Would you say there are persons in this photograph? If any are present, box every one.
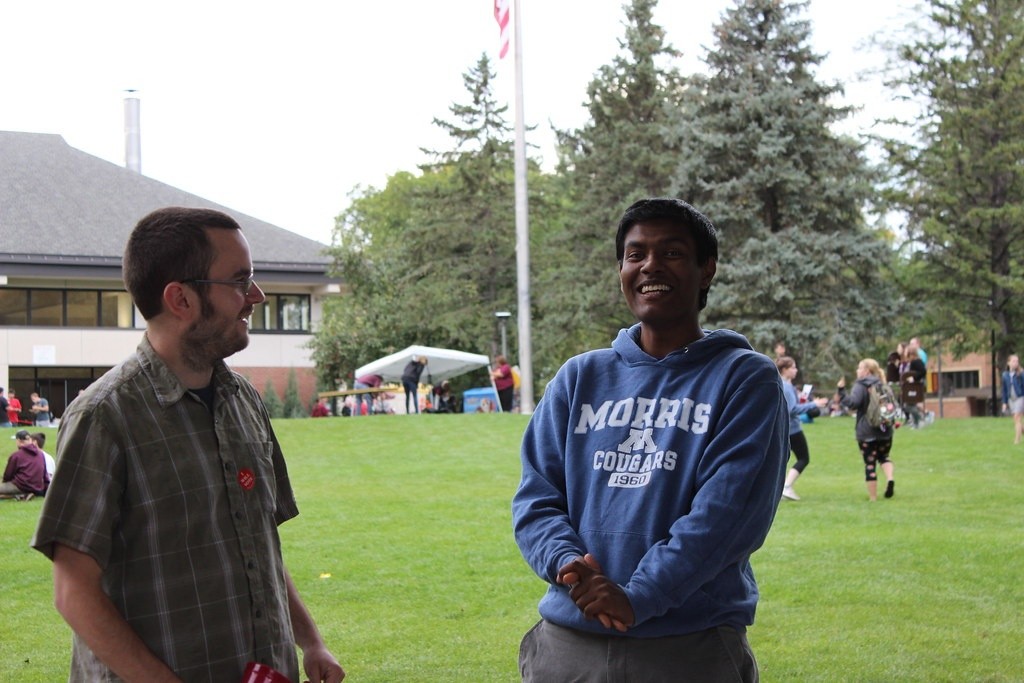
[355,375,383,416]
[512,198,788,683]
[887,338,935,429]
[31,393,50,426]
[829,395,846,416]
[401,357,427,414]
[0,430,55,500]
[33,207,347,683]
[838,359,894,501]
[773,343,827,499]
[313,397,332,417]
[0,387,11,427]
[433,380,450,410]
[489,356,514,412]
[1001,355,1024,443]
[6,389,22,426]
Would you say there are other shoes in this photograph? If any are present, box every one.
[885,480,895,498]
[783,487,799,501]
[909,421,921,430]
[927,412,935,425]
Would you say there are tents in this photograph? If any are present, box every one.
[355,345,503,412]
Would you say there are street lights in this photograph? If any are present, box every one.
[494,311,512,361]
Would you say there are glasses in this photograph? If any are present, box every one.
[185,274,253,296]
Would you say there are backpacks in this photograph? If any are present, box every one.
[865,382,899,433]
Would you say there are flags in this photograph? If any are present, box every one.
[493,0,510,60]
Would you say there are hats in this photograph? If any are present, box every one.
[12,430,31,441]
